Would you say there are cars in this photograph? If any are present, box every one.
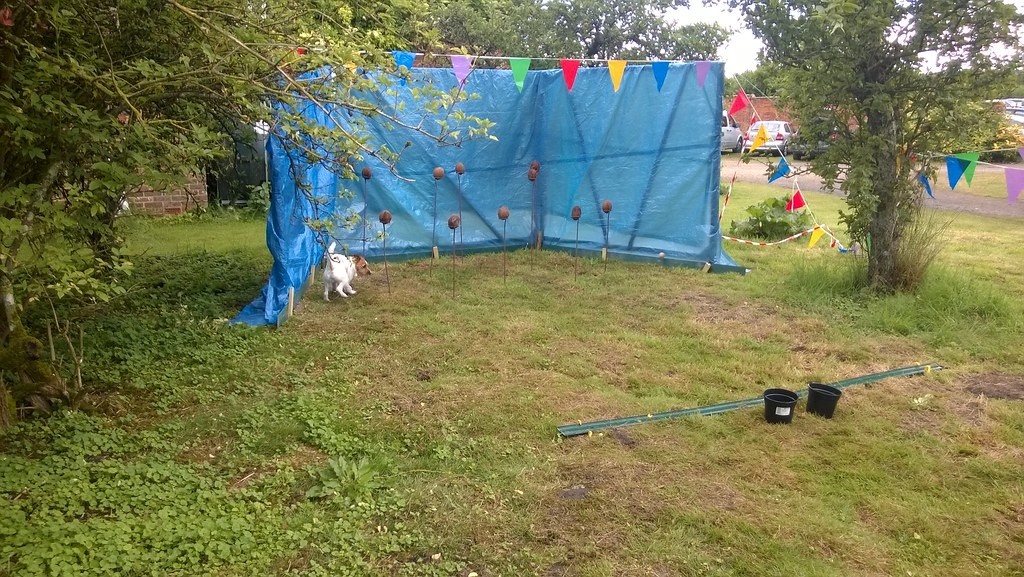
[985,98,1024,116]
[720,110,744,155]
[744,121,800,157]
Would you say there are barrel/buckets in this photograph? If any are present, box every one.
[764,388,799,424]
[806,384,842,419]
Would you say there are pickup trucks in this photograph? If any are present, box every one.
[792,117,853,160]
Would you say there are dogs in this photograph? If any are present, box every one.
[323,242,372,301]
